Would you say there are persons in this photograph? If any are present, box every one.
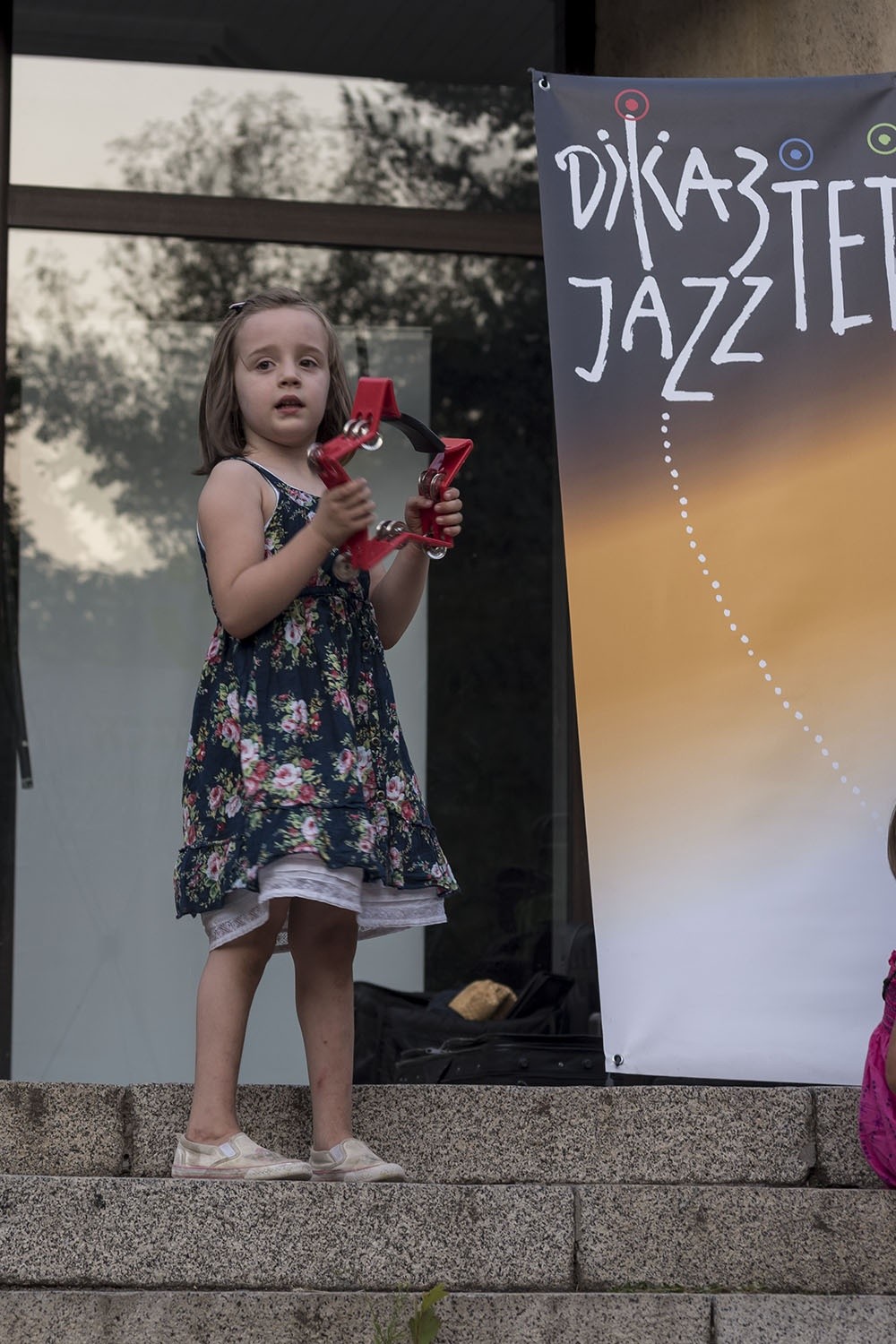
[170,285,462,1182]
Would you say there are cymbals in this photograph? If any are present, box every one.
[306,377,473,571]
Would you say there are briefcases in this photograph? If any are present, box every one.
[395,1032,826,1087]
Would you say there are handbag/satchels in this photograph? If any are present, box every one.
[353,969,590,1090]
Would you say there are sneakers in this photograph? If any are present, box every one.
[308,1138,406,1182]
[170,1133,313,1182]
